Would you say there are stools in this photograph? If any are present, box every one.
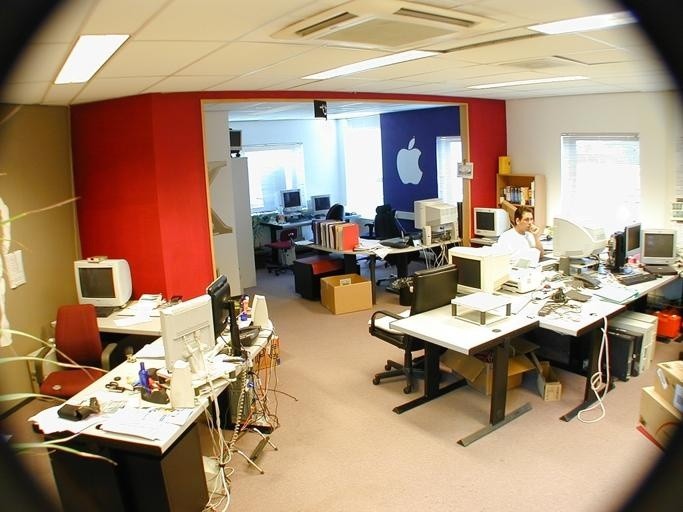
[263,241,291,276]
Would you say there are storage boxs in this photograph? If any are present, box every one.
[537,361,561,402]
[440,338,540,396]
[636,386,683,464]
[321,273,372,314]
[655,360,682,413]
[251,336,280,371]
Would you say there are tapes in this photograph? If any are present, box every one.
[150,389,159,396]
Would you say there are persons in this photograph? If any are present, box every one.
[498,206,544,260]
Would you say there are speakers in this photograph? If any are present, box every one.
[451,221,458,240]
[251,294,268,327]
[423,226,432,245]
[314,100,327,118]
[170,360,196,408]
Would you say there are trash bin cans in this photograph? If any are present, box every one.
[399,276,417,307]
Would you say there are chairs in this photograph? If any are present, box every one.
[368,263,458,394]
[326,204,345,221]
[365,204,413,285]
[34,304,120,400]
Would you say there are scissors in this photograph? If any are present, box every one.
[105,381,122,390]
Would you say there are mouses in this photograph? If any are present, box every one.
[656,274,662,277]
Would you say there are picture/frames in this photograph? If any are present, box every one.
[457,162,473,179]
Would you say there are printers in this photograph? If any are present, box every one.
[502,248,542,294]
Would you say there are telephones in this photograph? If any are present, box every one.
[573,273,602,290]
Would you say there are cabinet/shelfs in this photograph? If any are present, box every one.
[496,174,546,227]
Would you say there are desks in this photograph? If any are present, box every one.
[260,215,359,268]
[295,235,462,305]
[50,301,164,337]
[33,317,275,512]
[488,263,683,422]
[390,304,539,447]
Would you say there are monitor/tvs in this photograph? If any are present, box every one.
[414,197,457,232]
[205,274,246,359]
[640,228,677,265]
[229,129,242,151]
[553,217,608,259]
[279,189,302,213]
[307,194,331,216]
[448,245,512,294]
[73,258,132,308]
[474,207,510,241]
[616,223,641,273]
[160,294,217,379]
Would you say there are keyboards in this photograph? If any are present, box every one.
[96,305,113,318]
[621,273,657,287]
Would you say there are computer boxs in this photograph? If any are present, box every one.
[576,310,660,382]
[26,342,62,399]
[278,246,296,265]
